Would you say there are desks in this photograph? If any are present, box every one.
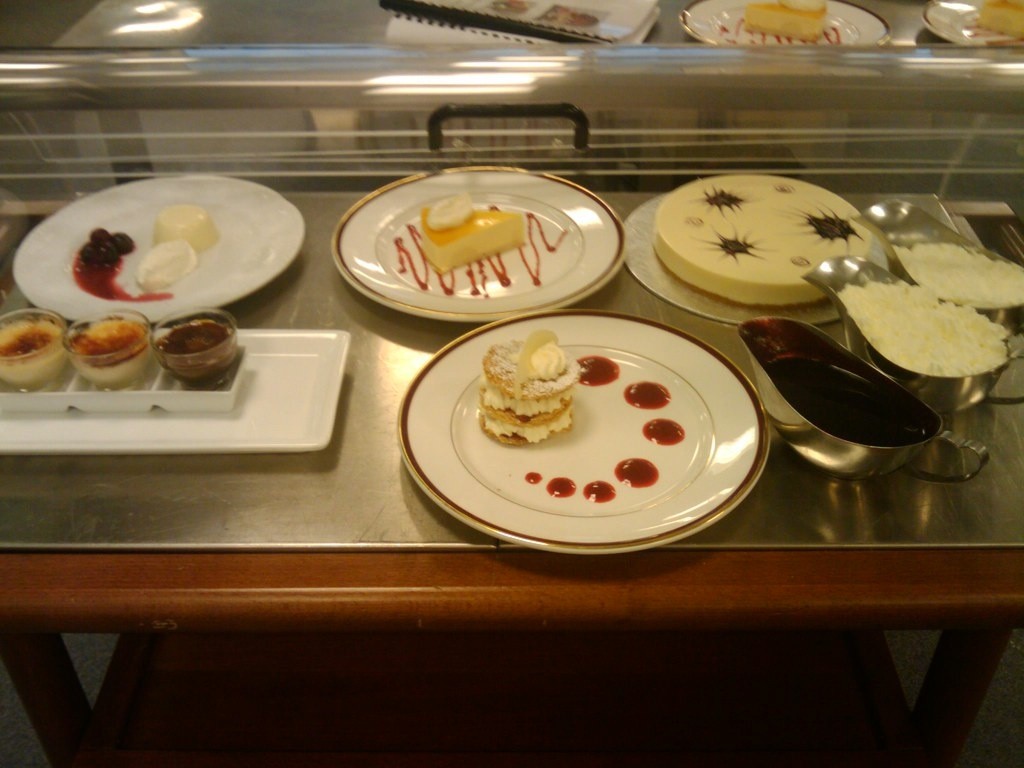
[1,549,1024,768]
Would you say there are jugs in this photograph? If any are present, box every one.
[737,317,989,483]
[851,199,1024,333]
[803,256,1024,426]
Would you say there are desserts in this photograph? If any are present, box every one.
[975,0,1024,40]
[479,328,580,446]
[136,204,219,290]
[417,192,527,275]
[745,0,829,43]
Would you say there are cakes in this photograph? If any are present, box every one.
[651,174,876,312]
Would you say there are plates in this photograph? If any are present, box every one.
[399,309,771,554]
[332,166,627,323]
[13,177,304,322]
[0,327,350,453]
[679,0,891,51]
[921,0,1024,47]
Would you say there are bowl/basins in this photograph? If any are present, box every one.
[61,308,150,389]
[151,306,237,385]
[0,308,67,387]
[0,344,246,413]
